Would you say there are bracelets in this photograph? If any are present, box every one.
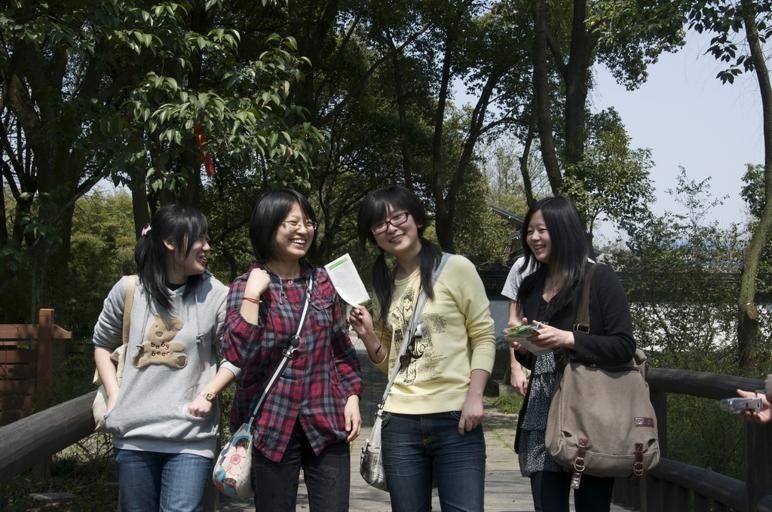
[242,296,263,305]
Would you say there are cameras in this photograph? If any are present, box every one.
[720,397,763,414]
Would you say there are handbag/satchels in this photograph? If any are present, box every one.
[89,343,129,428]
[544,260,661,480]
[359,409,441,494]
[209,420,256,501]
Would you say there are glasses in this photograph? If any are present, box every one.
[370,208,413,235]
[279,217,320,231]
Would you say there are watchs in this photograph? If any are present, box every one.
[200,390,216,404]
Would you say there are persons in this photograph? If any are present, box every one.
[346,183,497,512]
[506,195,636,512]
[219,186,363,512]
[498,252,595,402]
[92,205,241,512]
[735,373,772,430]
